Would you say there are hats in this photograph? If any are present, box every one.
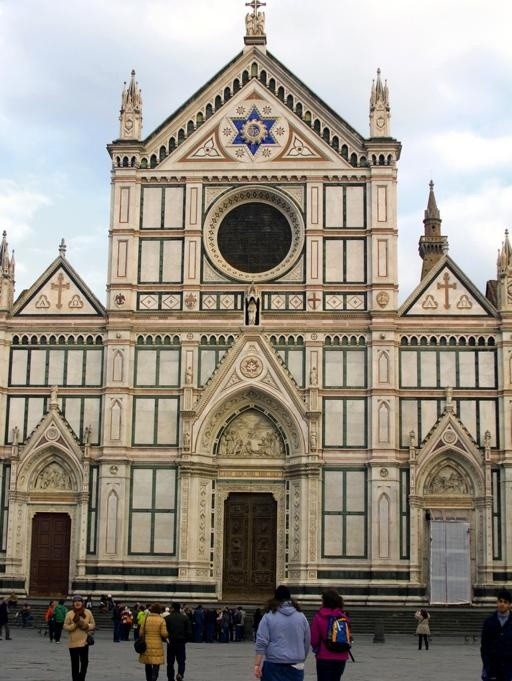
[73,595,82,601]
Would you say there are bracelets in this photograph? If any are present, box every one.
[255,663,262,667]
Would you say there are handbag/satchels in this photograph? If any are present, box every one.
[134,637,146,653]
[86,637,94,646]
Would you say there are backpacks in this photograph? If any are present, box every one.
[326,613,352,653]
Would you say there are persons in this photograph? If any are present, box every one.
[163,604,191,681]
[62,595,96,681]
[43,593,264,641]
[139,602,171,681]
[7,591,19,607]
[480,589,511,681]
[415,607,431,649]
[310,590,352,680]
[0,598,12,642]
[17,602,35,628]
[252,585,311,681]
